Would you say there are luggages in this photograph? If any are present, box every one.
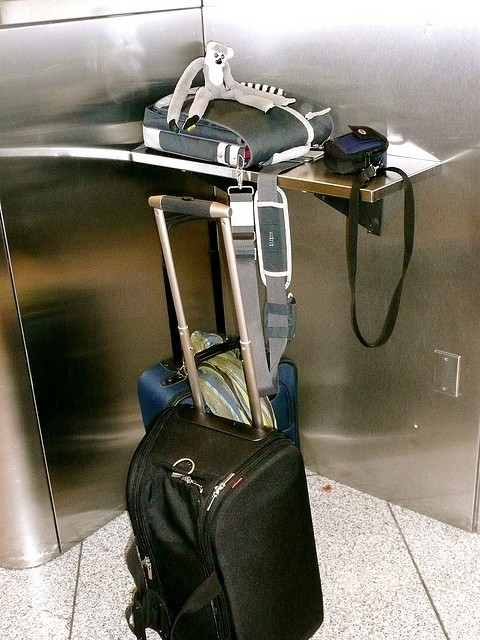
[126,195,324,639]
[137,210,300,449]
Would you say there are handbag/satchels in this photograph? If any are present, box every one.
[141,83,333,165]
[323,125,390,174]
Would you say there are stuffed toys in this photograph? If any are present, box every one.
[166,42,286,133]
